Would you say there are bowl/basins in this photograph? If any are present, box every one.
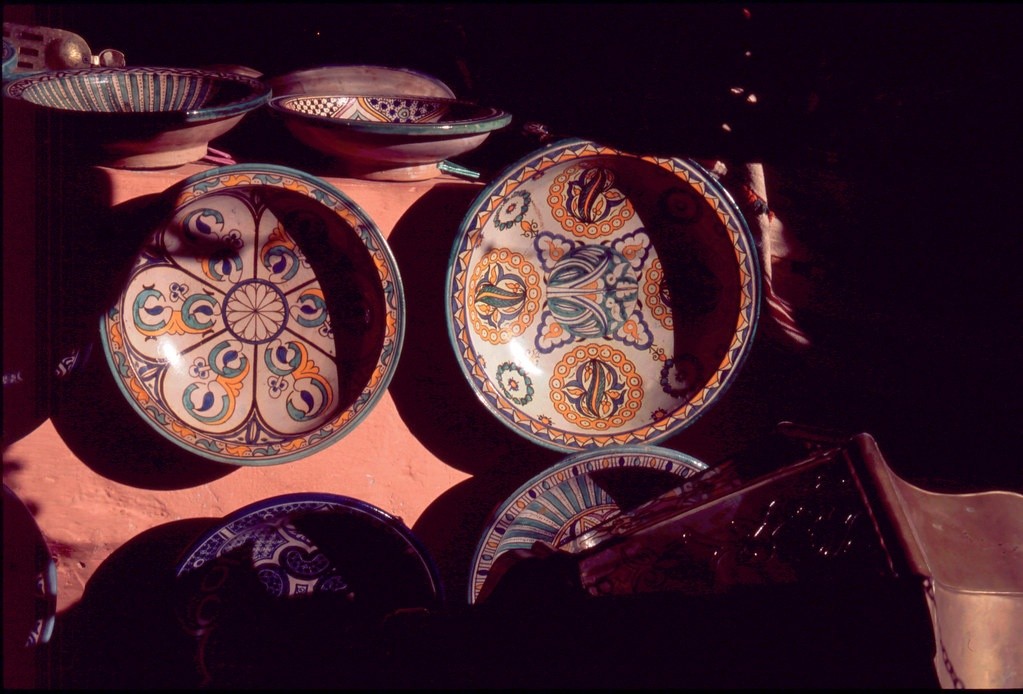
[1,65,271,170]
[273,64,456,99]
[1,480,54,693]
[269,91,514,184]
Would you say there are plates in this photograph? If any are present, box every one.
[99,162,407,468]
[167,492,444,694]
[444,138,763,455]
[466,443,711,605]
